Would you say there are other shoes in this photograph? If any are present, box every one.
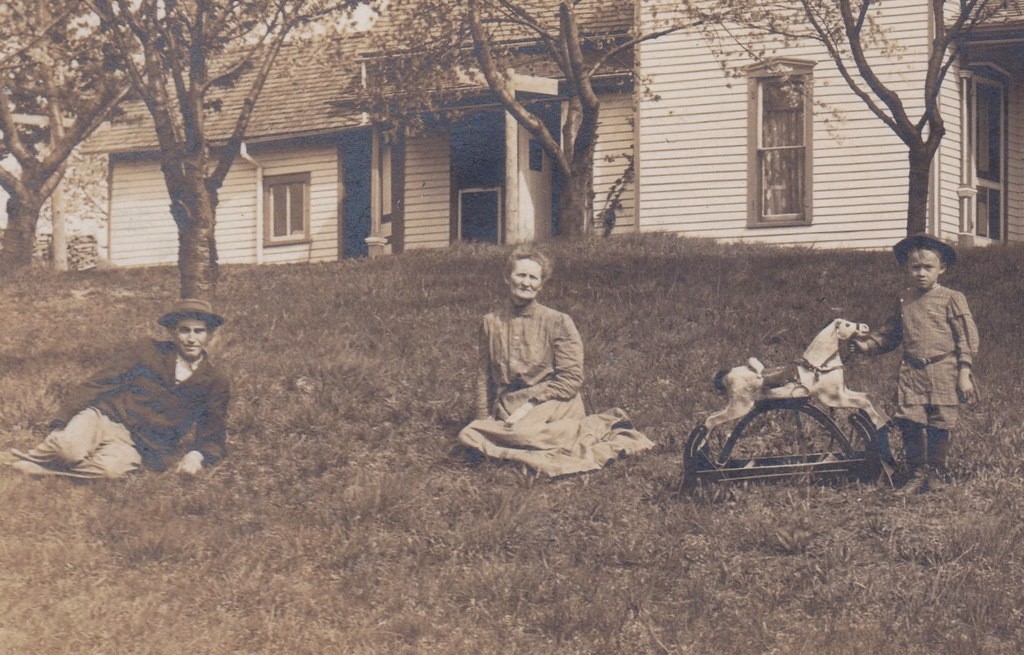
[924,463,949,496]
[897,475,925,494]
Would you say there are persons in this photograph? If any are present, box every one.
[455,249,656,477]
[0,300,230,478]
[848,233,979,496]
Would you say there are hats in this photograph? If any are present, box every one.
[893,232,956,267]
[157,298,224,327]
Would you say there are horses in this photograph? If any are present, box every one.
[697,317,885,449]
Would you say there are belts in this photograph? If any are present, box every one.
[903,348,959,369]
